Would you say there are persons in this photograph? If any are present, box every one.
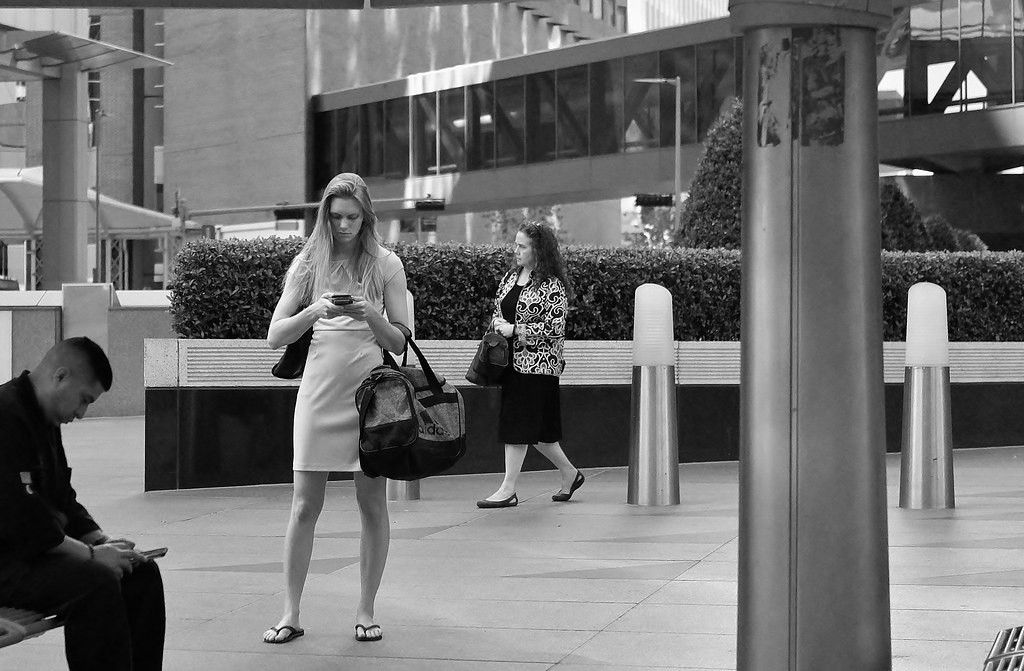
[0,336,166,671]
[473,219,588,511]
[260,171,409,638]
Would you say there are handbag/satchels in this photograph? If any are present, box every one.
[271,253,314,380]
[355,322,465,482]
[465,318,509,387]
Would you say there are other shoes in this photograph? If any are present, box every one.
[552,469,585,502]
[477,492,518,508]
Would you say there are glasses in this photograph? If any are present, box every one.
[531,220,543,239]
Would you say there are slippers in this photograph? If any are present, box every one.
[263,626,304,643]
[355,623,382,641]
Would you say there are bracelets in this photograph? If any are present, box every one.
[512,323,517,336]
[93,535,110,546]
[83,543,95,565]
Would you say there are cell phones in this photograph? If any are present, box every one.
[332,295,353,305]
[131,548,168,563]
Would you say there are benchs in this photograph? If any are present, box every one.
[983,626,1024,671]
[0,605,64,648]
